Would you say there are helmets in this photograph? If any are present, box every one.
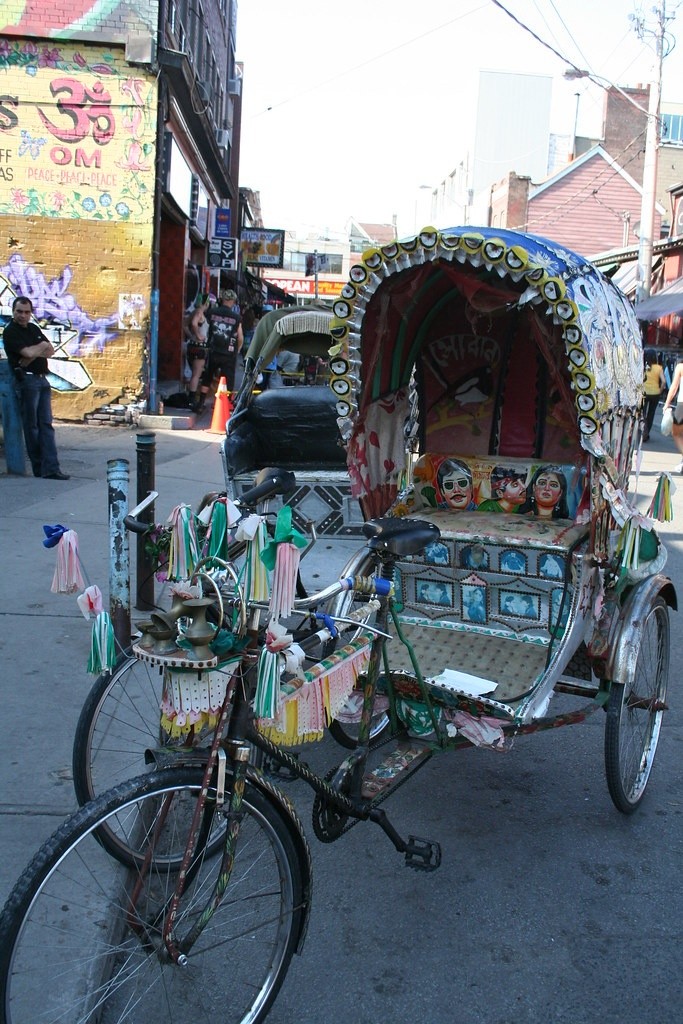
[261,304,273,312]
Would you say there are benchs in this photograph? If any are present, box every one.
[226,383,386,538]
[393,448,595,580]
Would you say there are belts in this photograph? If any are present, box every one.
[25,371,44,378]
[189,341,207,348]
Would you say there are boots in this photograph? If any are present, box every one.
[185,391,201,414]
[198,392,206,409]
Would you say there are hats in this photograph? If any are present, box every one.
[222,289,237,301]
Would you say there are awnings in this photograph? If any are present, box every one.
[635,276,683,319]
[610,254,662,296]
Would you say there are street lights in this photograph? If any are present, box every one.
[565,66,661,308]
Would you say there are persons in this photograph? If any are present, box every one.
[663,360,683,452]
[243,305,327,391]
[3,296,72,480]
[641,349,666,442]
[197,289,244,411]
[184,294,209,405]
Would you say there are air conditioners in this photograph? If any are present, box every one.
[197,81,214,102]
[227,80,241,97]
[213,129,229,151]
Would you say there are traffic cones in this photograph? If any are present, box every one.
[203,376,232,436]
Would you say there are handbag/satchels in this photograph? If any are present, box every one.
[660,408,673,437]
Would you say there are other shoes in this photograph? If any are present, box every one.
[43,471,71,480]
[643,435,649,443]
[34,473,41,477]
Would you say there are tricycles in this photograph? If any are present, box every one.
[0,225,678,1023]
[71,299,392,873]
